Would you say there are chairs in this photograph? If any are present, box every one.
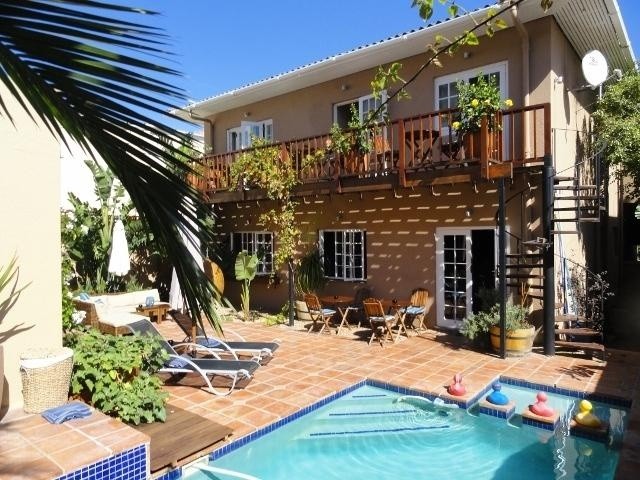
[302,294,337,337]
[166,310,278,365]
[126,318,261,396]
[362,299,395,348]
[341,287,370,329]
[399,287,429,337]
[369,127,464,172]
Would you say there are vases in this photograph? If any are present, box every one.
[463,132,483,166]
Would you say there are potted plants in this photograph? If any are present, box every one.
[457,302,533,358]
[292,248,327,320]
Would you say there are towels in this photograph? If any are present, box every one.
[42,401,91,424]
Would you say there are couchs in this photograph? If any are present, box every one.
[73,288,160,339]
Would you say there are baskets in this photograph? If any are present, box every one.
[20,345,75,415]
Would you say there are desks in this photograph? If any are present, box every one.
[318,295,355,334]
[371,297,411,333]
[134,305,171,323]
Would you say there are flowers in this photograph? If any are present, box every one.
[450,71,514,133]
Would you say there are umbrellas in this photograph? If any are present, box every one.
[109,219,131,279]
[170,196,205,344]
